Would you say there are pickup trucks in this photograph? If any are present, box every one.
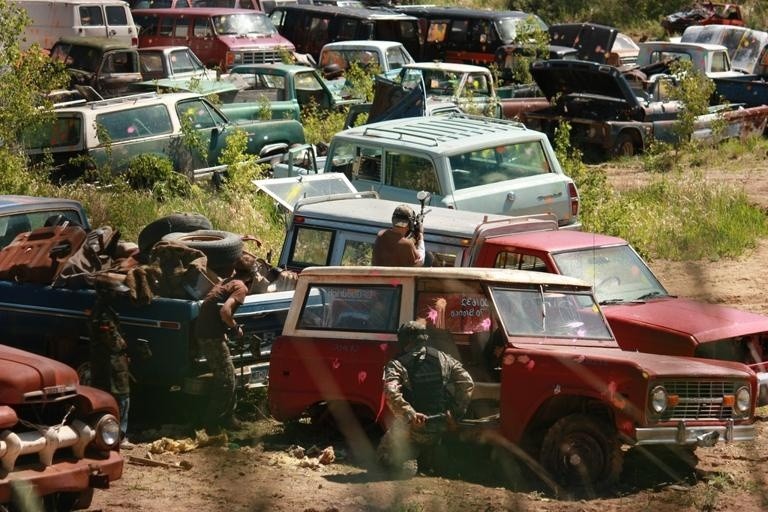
[1,192,331,419]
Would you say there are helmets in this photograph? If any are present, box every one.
[397,320,429,351]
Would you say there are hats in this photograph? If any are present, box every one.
[393,204,414,229]
[96,275,130,294]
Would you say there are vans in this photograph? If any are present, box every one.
[269,112,584,234]
[0,2,768,191]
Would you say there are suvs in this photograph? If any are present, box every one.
[0,340,127,512]
[265,265,761,494]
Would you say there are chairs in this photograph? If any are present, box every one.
[427,328,501,415]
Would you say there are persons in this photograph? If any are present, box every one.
[374,321,474,479]
[487,328,505,383]
[87,288,130,440]
[195,254,255,430]
[372,206,426,267]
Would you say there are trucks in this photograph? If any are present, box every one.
[248,173,767,409]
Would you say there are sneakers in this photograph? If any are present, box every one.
[119,438,134,449]
[225,417,251,430]
[400,445,421,480]
[212,426,236,437]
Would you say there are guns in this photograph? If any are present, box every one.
[416,210,432,225]
